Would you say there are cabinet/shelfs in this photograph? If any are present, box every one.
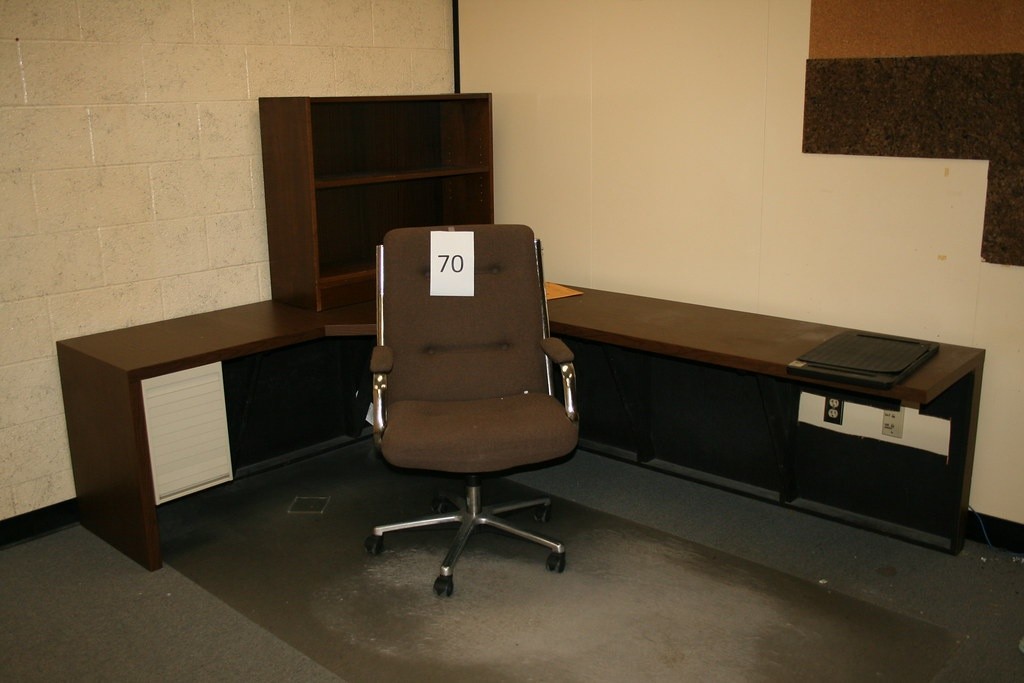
[260,92,494,313]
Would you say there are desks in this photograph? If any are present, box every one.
[55,282,986,573]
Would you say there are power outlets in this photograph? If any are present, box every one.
[823,395,845,426]
[881,405,906,439]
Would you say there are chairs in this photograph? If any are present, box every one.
[364,225,581,600]
